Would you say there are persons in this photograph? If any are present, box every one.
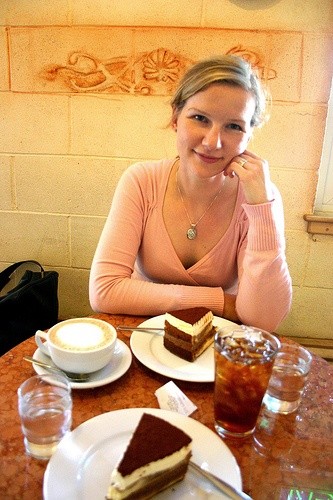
[89,57,292,333]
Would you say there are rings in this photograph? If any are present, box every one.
[239,159,248,167]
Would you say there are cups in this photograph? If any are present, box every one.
[262,342,313,415]
[35,317,117,373]
[213,324,281,441]
[17,375,73,460]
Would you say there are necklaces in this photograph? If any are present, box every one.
[175,164,225,241]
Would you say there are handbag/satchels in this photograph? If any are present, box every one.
[0,260,59,356]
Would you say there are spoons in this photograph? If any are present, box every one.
[24,356,90,380]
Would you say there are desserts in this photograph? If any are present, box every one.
[104,412,193,500]
[163,306,218,362]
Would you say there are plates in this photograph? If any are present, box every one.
[130,315,240,383]
[32,339,132,390]
[43,408,242,500]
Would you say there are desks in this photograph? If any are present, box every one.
[0,312,333,500]
